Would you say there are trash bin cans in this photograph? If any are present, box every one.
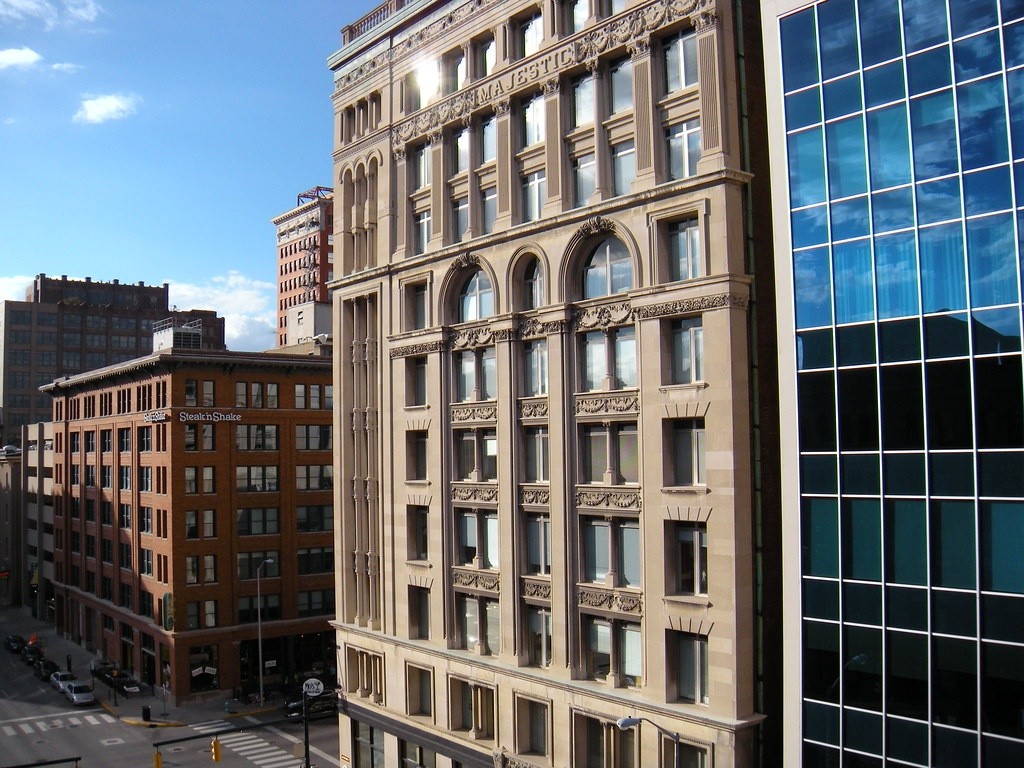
[141,706,151,722]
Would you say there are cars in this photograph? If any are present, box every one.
[64,681,94,706]
[285,691,339,718]
[5,635,25,653]
[19,645,44,666]
[33,659,60,681]
[50,670,80,693]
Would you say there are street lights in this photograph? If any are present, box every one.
[617,716,681,768]
[257,558,273,707]
